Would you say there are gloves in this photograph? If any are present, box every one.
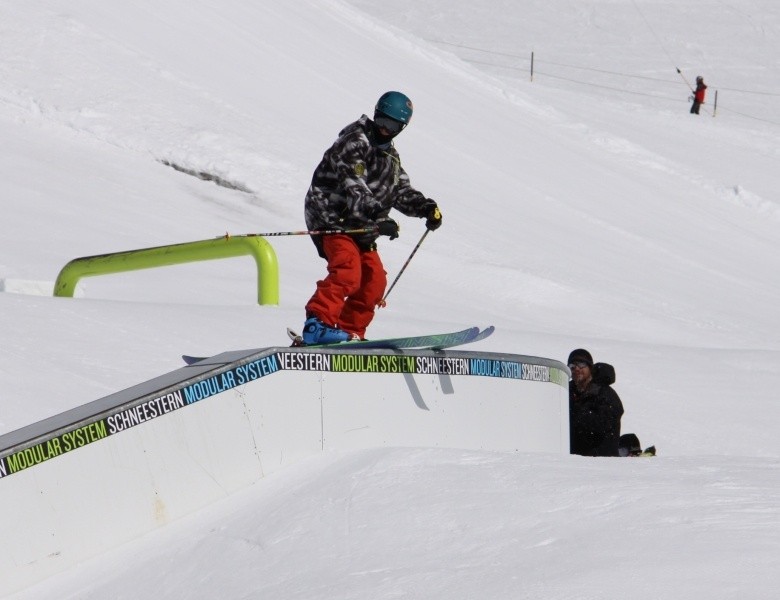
[375,216,400,240]
[424,205,443,232]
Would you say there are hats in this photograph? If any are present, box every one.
[568,348,593,368]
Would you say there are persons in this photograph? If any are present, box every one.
[690,76,705,113]
[300,92,447,345]
[570,348,623,458]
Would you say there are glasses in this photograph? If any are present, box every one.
[568,361,588,368]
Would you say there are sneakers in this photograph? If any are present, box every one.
[303,318,353,345]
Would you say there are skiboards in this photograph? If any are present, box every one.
[181,323,496,364]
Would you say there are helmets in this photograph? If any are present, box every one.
[375,90,414,124]
[696,75,703,81]
